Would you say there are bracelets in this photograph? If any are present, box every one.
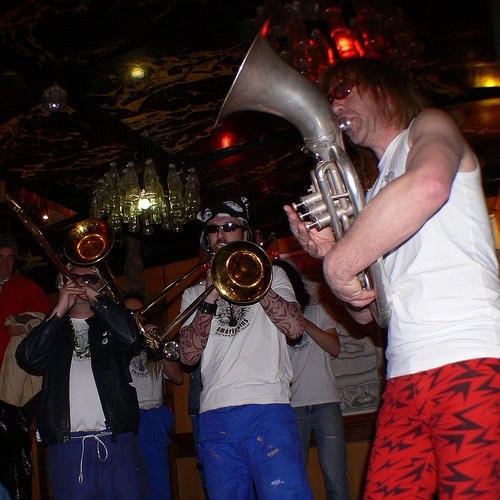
[196,300,218,315]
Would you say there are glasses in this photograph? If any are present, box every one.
[126,307,144,317]
[205,222,246,234]
[326,79,368,104]
[65,273,104,285]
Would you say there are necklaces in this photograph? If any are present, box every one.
[68,314,92,359]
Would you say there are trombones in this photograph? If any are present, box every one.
[4,193,125,306]
[129,241,274,350]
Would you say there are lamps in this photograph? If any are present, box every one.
[87,159,201,235]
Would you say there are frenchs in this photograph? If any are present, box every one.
[211,30,392,330]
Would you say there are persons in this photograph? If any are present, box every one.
[0,196,349,500]
[283,55,500,500]
